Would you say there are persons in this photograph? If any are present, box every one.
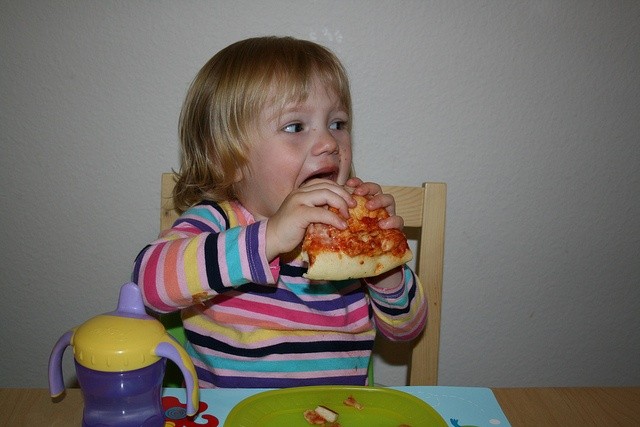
[130,36,428,389]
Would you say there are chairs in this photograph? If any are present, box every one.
[159,172,448,386]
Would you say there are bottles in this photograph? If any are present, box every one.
[46,283,197,425]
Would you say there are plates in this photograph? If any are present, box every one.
[223,386,448,426]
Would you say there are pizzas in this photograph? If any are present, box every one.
[299,195,413,281]
